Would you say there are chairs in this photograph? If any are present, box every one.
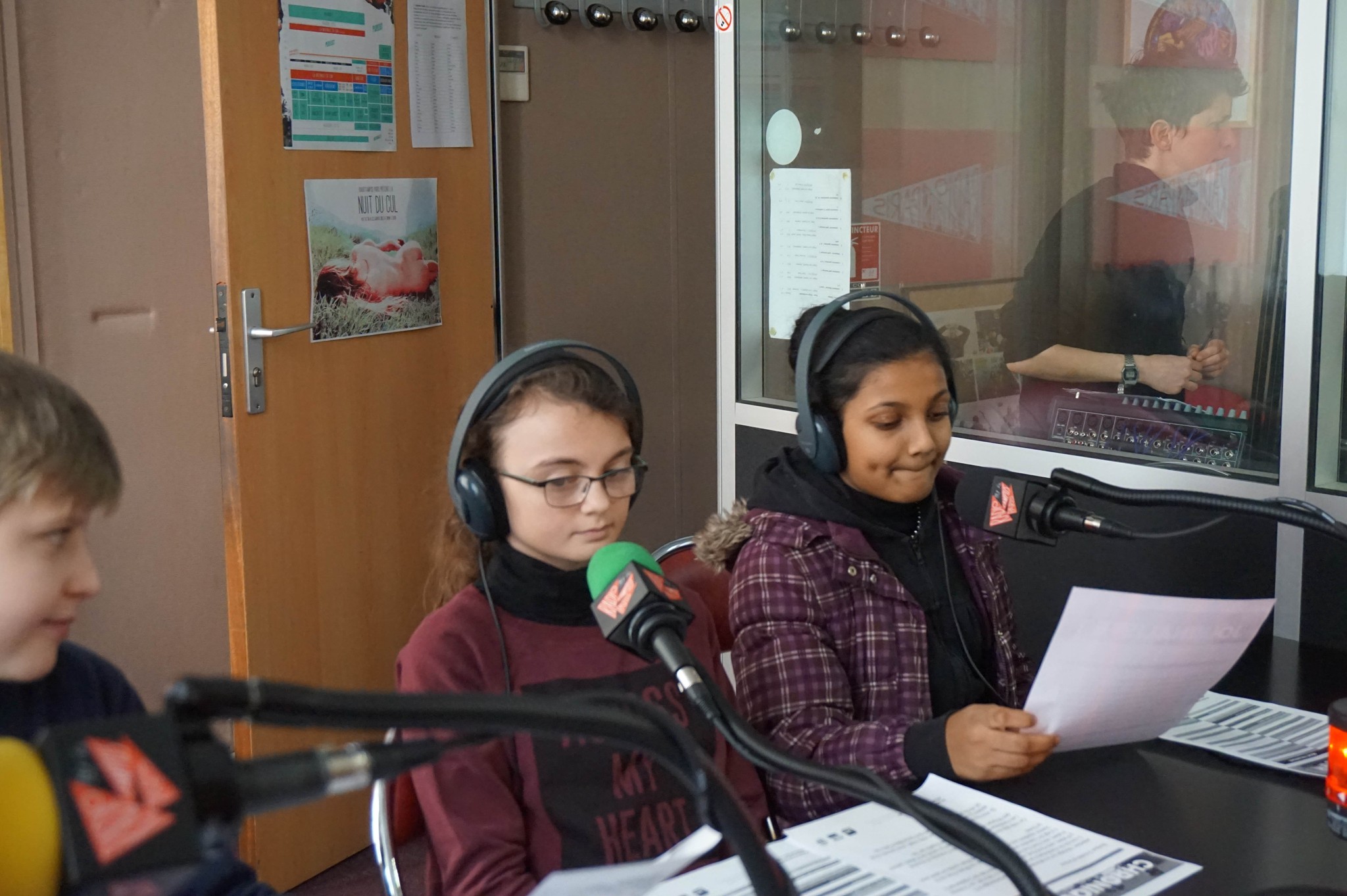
[653,522,736,656]
[367,726,447,896]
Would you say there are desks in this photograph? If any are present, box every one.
[967,686,1347,896]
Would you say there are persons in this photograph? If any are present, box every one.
[392,353,765,896]
[689,302,1060,826]
[317,232,437,318]
[998,49,1249,441]
[0,346,281,896]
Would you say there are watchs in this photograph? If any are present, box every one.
[1122,353,1140,389]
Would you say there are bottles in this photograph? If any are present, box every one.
[1326,697,1347,841]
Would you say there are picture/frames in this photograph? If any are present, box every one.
[899,275,1023,437]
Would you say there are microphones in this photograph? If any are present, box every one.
[0,714,444,896]
[585,541,723,725]
[953,469,1134,548]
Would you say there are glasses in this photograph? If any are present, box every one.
[496,453,649,508]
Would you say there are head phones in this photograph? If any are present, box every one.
[795,290,958,475]
[446,340,643,542]
